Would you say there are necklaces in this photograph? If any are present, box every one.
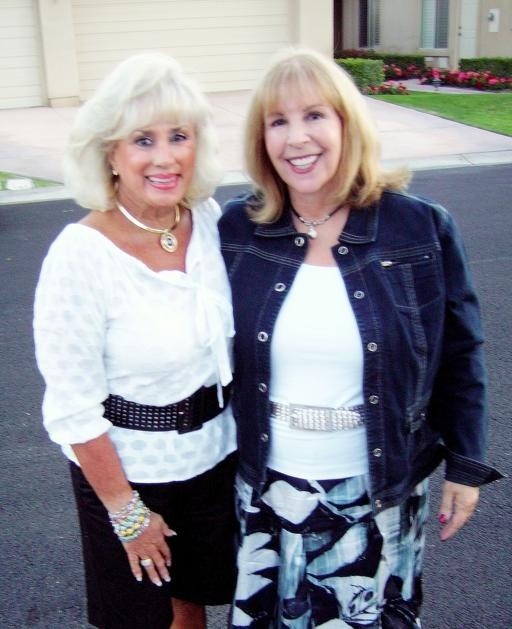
[290,198,347,241]
[111,198,182,254]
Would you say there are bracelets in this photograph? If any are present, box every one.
[104,489,151,541]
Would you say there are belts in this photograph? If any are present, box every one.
[101,380,233,436]
[268,399,367,433]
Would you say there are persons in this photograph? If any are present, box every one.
[211,49,510,628]
[32,62,241,628]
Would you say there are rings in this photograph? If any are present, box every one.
[139,558,153,567]
